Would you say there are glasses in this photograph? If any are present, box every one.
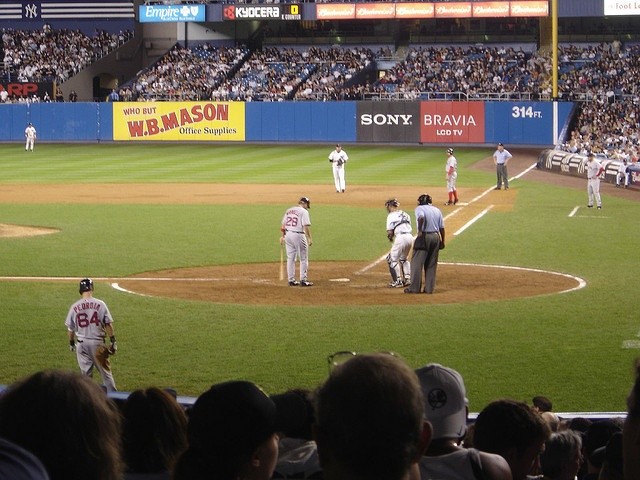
[327,349,398,378]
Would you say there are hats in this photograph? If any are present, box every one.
[414,362,469,441]
[587,153,593,157]
[187,381,307,477]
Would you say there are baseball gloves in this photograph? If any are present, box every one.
[336,157,344,165]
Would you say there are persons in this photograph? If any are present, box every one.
[584,420,622,480]
[275,388,325,479]
[281,197,313,286]
[616,158,631,188]
[1,20,132,102]
[66,277,118,393]
[533,396,552,414]
[445,148,459,206]
[542,412,559,432]
[25,123,36,152]
[557,37,640,102]
[403,195,445,294]
[329,144,349,193]
[105,42,553,101]
[623,359,640,479]
[610,418,624,432]
[169,382,280,480]
[404,362,511,480]
[565,104,640,153]
[318,355,434,480]
[493,142,513,190]
[605,153,640,162]
[570,418,591,432]
[474,399,551,479]
[585,153,604,210]
[591,431,624,480]
[543,431,584,480]
[1,370,123,480]
[111,387,187,479]
[556,420,570,432]
[384,198,415,288]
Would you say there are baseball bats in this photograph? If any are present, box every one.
[280,238,284,283]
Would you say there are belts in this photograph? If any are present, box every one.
[286,230,304,234]
[422,231,437,234]
[401,232,407,234]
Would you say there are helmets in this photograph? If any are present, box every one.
[300,197,310,209]
[336,144,341,147]
[80,278,93,291]
[385,199,399,207]
[447,148,453,155]
[417,194,432,205]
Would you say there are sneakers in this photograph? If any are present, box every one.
[597,206,601,209]
[444,201,453,206]
[404,288,413,293]
[505,187,509,191]
[387,280,404,288]
[494,187,501,190]
[454,199,458,205]
[424,287,432,294]
[402,279,411,286]
[300,281,314,286]
[288,279,300,285]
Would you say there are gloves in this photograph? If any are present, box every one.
[70,344,77,352]
[108,342,117,355]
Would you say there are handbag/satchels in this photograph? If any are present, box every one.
[414,232,426,250]
